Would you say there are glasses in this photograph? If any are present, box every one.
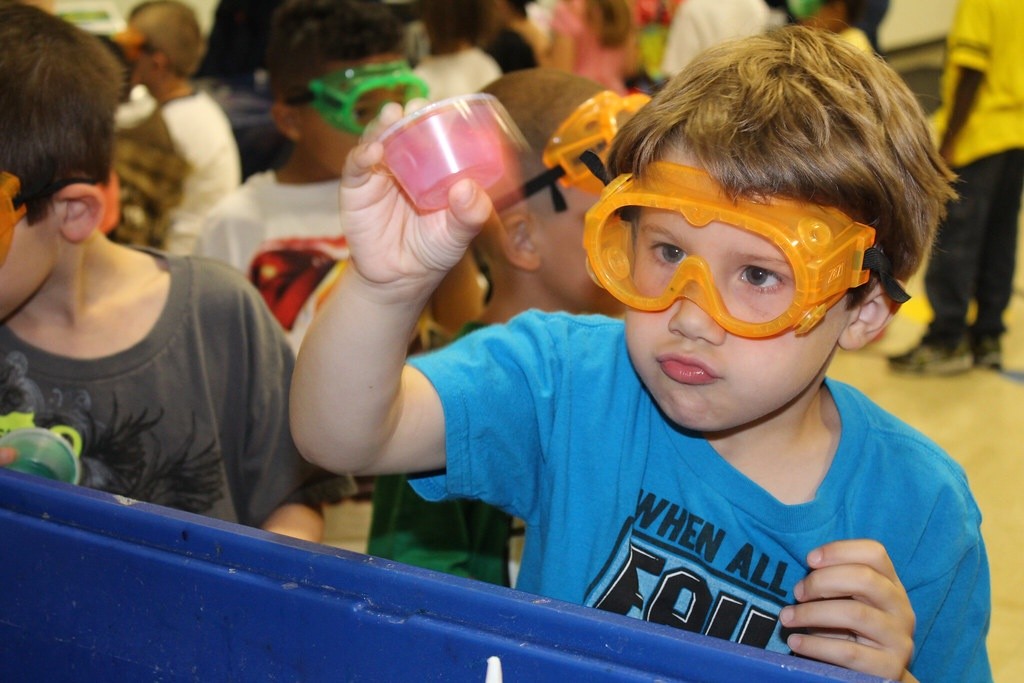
[582,162,911,339]
[492,91,651,215]
[284,58,429,135]
[112,29,191,80]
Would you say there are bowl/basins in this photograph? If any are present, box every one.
[372,92,505,211]
[0,428,80,484]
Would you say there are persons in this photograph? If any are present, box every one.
[289,22,995,683]
[0,0,357,546]
[1,0,1023,377]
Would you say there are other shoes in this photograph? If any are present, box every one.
[891,333,1001,373]
